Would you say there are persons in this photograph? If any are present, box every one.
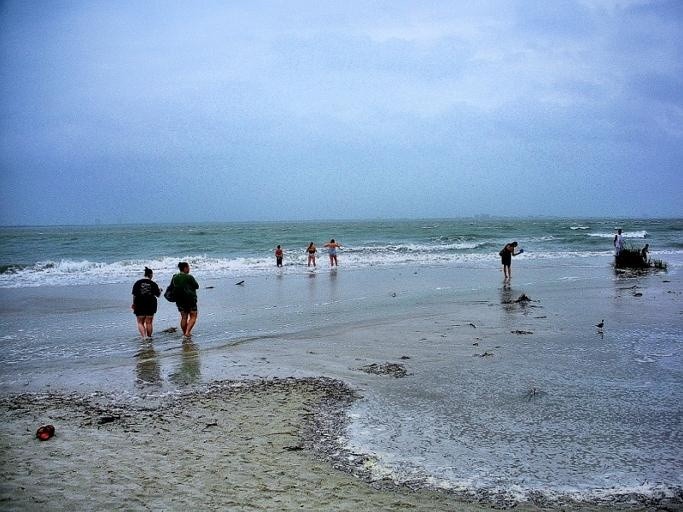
[305,242,317,267]
[133,339,161,387]
[167,338,200,386]
[641,244,649,263]
[499,242,523,278]
[613,228,627,269]
[131,266,163,338]
[503,278,512,291]
[171,262,199,337]
[274,244,283,267]
[323,239,340,266]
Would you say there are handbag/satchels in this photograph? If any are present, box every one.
[164,274,178,302]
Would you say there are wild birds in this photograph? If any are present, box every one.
[593,319,605,332]
